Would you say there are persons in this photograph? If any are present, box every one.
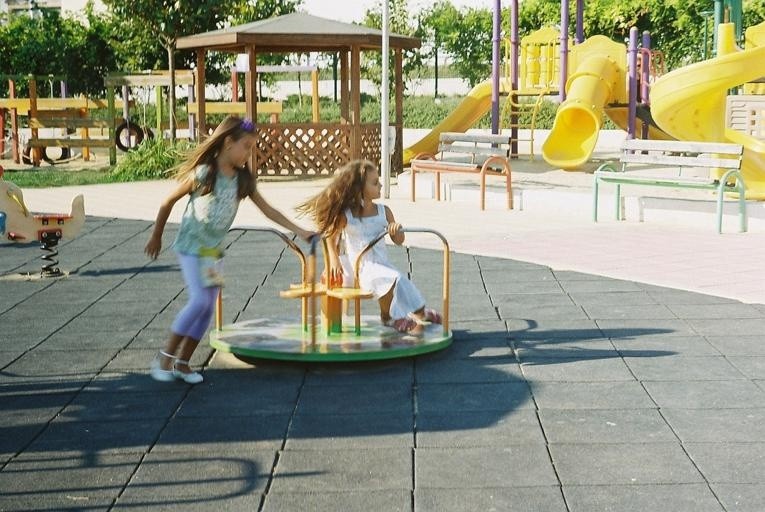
[295,159,444,333]
[634,56,642,103]
[143,113,321,385]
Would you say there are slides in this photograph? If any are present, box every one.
[402,21,765,199]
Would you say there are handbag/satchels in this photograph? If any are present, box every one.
[197,254,225,290]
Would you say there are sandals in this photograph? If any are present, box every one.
[382,315,417,332]
[420,308,443,324]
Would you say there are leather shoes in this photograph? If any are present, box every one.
[173,359,204,384]
[150,350,176,384]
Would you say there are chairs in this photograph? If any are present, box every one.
[0,165,86,280]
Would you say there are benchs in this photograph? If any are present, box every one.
[409,132,514,210]
[593,140,747,233]
